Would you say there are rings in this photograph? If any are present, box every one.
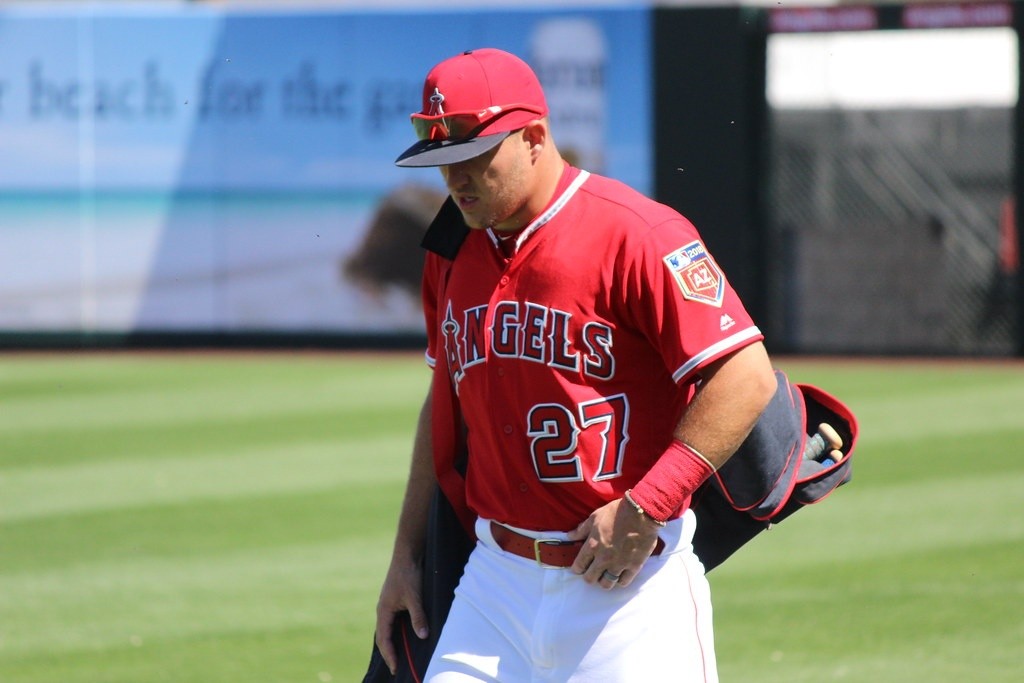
[603,571,620,583]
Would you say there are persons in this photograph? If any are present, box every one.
[372,46,780,683]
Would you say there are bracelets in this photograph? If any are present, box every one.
[624,488,666,527]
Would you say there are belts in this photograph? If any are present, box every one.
[489,521,666,566]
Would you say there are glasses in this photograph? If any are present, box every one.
[410,103,543,142]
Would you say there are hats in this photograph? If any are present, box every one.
[395,49,549,167]
